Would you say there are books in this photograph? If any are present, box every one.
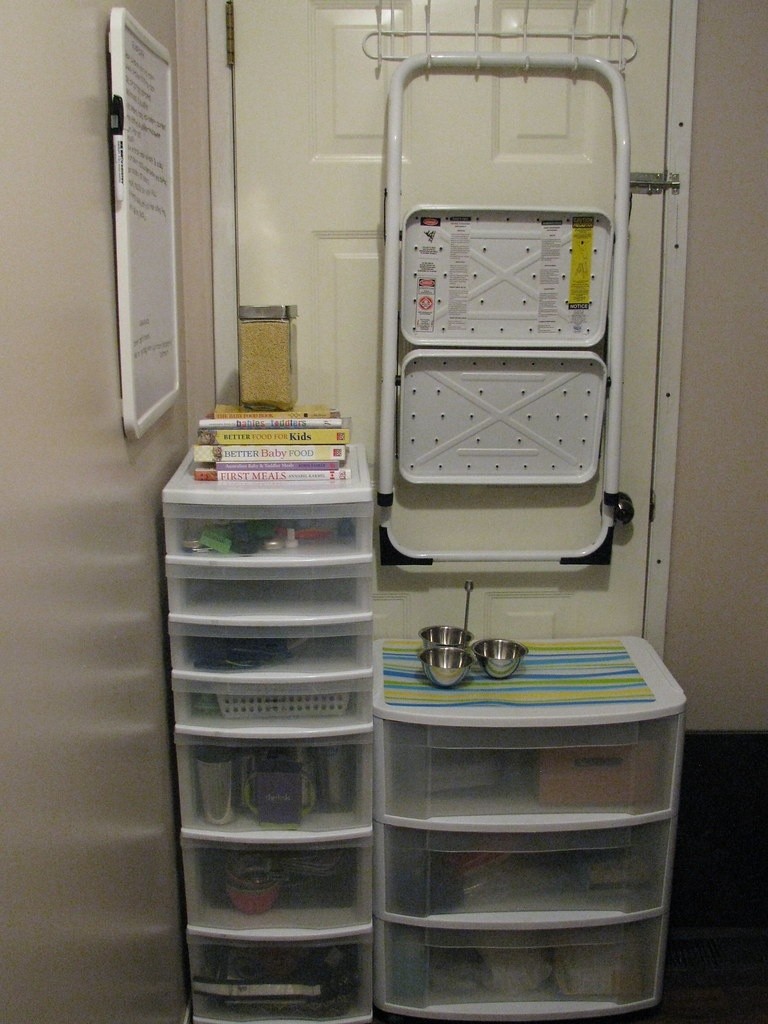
[195,404,353,482]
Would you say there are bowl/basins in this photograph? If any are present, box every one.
[472,638,529,679]
[224,863,277,912]
[417,646,476,688]
[417,625,475,651]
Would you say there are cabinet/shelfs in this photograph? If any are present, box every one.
[373,638,686,1021]
[161,444,377,1024]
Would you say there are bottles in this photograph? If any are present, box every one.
[238,305,298,412]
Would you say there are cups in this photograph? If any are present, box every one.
[194,748,241,826]
[316,744,355,813]
